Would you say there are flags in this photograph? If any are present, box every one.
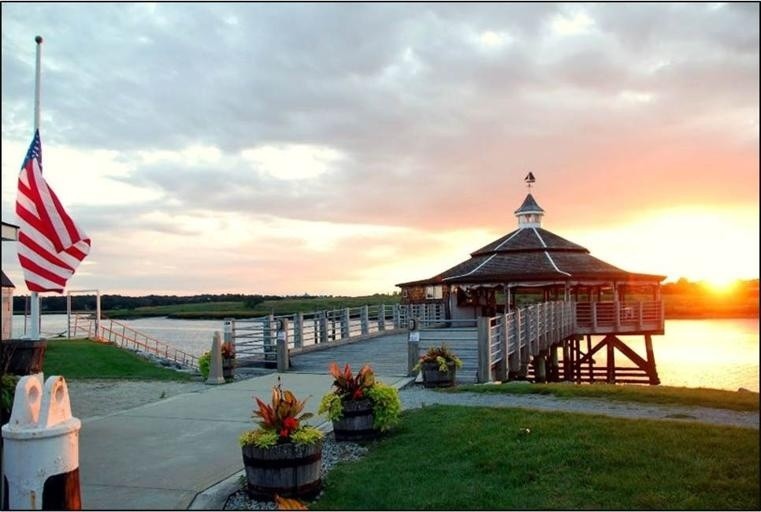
[16,128,93,294]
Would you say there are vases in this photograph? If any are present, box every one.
[421,362,455,389]
[221,358,234,383]
[332,401,372,442]
[241,443,322,502]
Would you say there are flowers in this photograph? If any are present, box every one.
[220,342,238,369]
[411,340,462,373]
[238,375,324,445]
[316,362,400,431]
